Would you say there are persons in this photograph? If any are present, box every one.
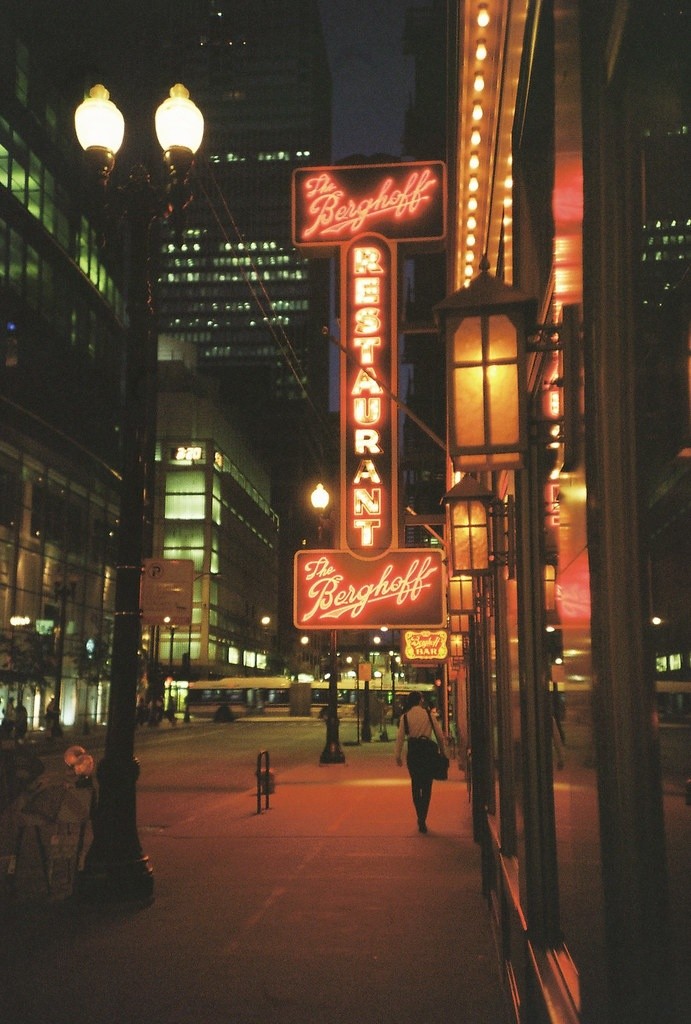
[395,692,446,832]
[0,698,178,743]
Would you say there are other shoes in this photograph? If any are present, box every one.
[418,819,427,832]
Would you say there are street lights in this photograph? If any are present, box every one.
[348,664,360,743]
[309,481,347,764]
[81,636,95,735]
[74,81,205,916]
[373,671,382,732]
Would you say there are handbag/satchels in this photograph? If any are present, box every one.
[429,751,450,781]
[408,735,439,768]
